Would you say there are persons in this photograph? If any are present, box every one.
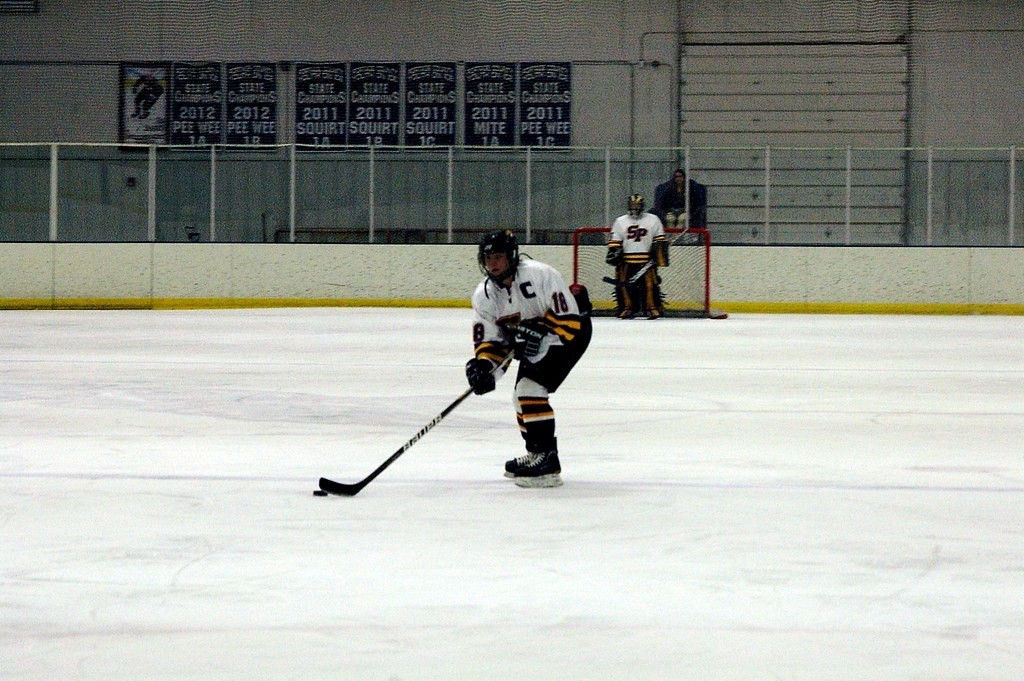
[464,229,592,487]
[664,169,690,241]
[607,195,669,321]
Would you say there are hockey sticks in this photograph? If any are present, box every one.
[602,232,686,287]
[319,348,515,498]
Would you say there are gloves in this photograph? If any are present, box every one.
[510,319,550,359]
[464,358,497,396]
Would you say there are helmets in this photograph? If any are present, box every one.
[475,229,519,280]
[627,193,645,215]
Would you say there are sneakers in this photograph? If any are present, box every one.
[504,451,538,478]
[514,436,563,488]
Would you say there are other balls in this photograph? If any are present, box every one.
[313,490,327,496]
